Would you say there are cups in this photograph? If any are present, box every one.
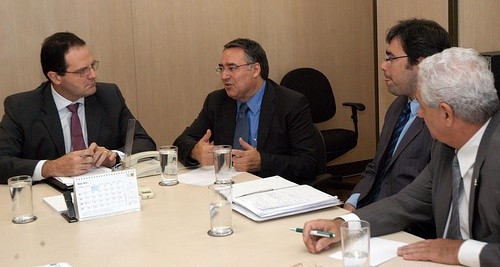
[158,145,178,186]
[340,219,371,267]
[207,183,233,236]
[212,145,233,185]
[7,175,34,224]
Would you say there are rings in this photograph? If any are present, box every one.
[107,150,110,153]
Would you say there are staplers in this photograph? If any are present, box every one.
[61,191,79,224]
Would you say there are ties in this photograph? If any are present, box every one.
[233,103,249,150]
[66,103,87,150]
[446,156,462,240]
[367,102,411,204]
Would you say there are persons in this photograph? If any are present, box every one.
[171,37,324,185]
[344,18,451,240]
[303,46,500,267]
[0,32,156,185]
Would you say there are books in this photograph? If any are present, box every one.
[44,166,113,191]
[215,175,343,222]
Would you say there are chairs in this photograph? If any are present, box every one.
[279,68,364,190]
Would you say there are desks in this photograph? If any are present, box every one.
[0,160,465,267]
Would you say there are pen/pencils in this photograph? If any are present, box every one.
[80,155,87,159]
[289,228,335,238]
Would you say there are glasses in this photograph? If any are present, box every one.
[215,63,256,74]
[384,56,408,61]
[58,60,98,77]
